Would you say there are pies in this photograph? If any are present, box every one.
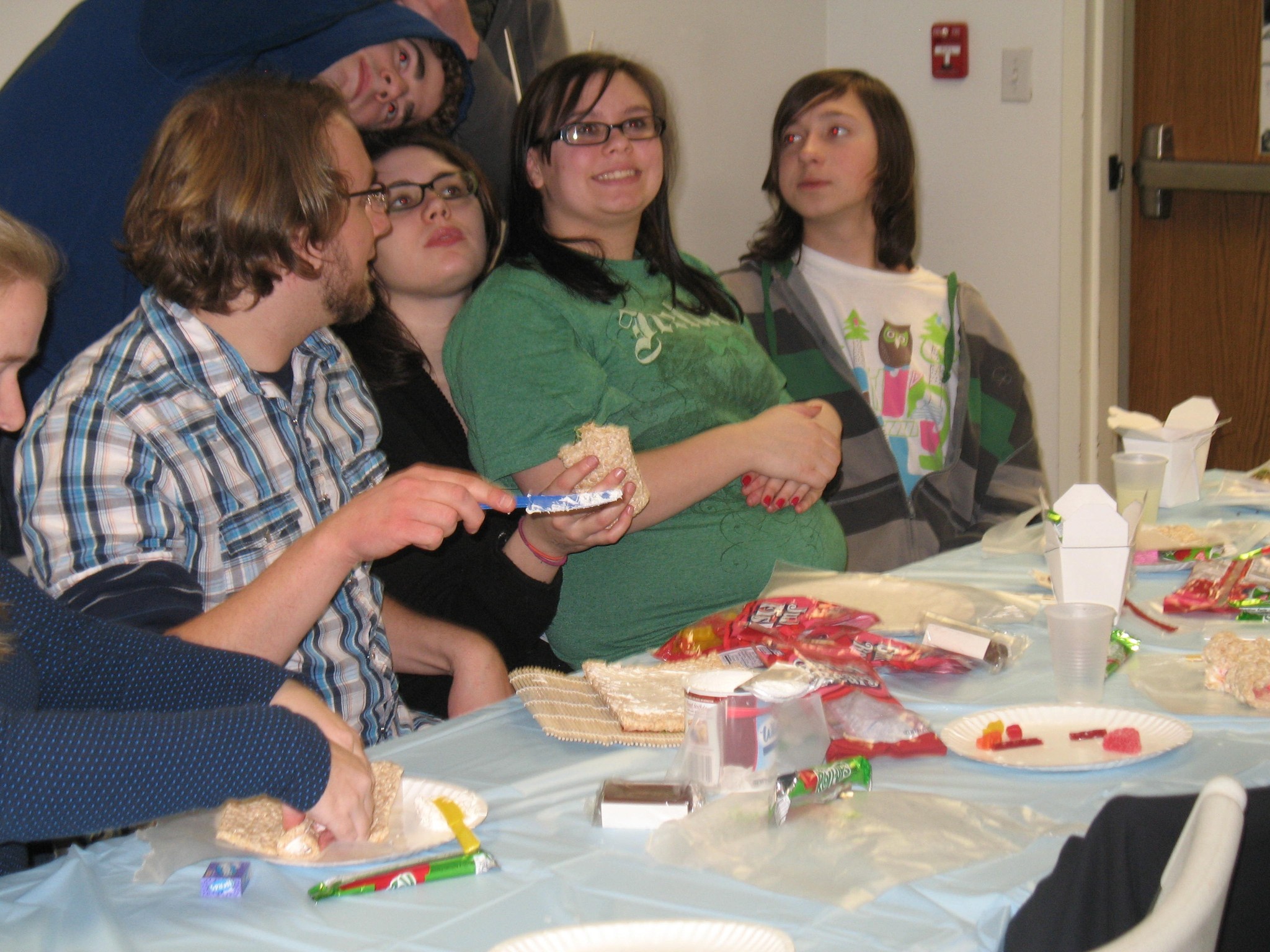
[1204,630,1270,712]
[217,761,405,861]
[582,652,739,731]
[1132,524,1206,548]
[558,423,650,529]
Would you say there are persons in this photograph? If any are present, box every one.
[714,68,1052,573]
[0,0,638,847]
[438,53,849,667]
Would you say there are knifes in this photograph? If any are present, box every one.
[476,489,622,512]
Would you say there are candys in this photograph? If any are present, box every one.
[978,721,1142,755]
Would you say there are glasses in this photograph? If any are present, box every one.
[384,169,478,212]
[533,116,668,148]
[340,183,389,215]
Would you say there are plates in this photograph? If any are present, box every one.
[1131,658,1242,716]
[937,705,1194,773]
[268,778,490,867]
[766,578,970,634]
[1136,545,1237,573]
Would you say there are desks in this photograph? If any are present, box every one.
[0,469,1270,952]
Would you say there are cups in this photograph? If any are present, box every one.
[1043,602,1118,701]
[1111,453,1170,522]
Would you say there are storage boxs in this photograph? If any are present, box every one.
[1033,480,1147,630]
[1105,393,1234,510]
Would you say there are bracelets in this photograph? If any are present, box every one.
[514,513,570,568]
